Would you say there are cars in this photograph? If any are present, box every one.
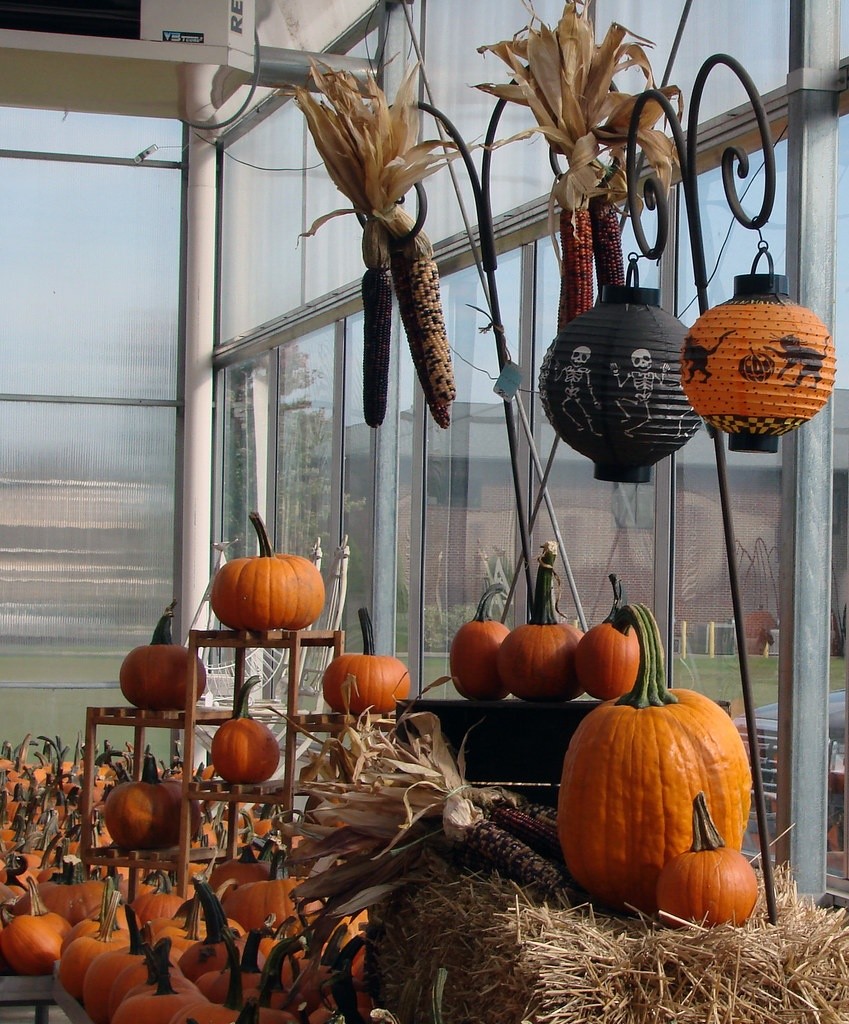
[732,687,849,872]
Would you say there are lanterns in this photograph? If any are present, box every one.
[541,285,701,485]
[677,274,838,456]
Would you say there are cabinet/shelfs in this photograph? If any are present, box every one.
[81,627,345,915]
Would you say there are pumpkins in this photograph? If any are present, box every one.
[0,512,758,1024]
